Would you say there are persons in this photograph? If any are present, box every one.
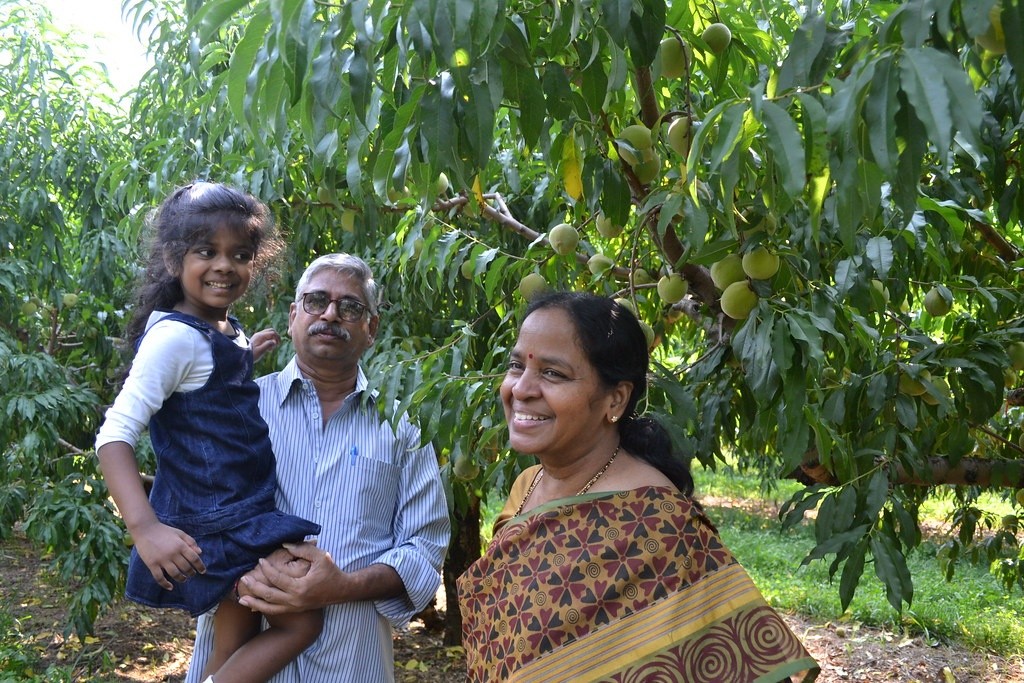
[455,288,822,683]
[96,185,333,683]
[187,251,453,683]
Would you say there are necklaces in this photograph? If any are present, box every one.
[512,441,622,518]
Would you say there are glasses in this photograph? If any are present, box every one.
[295,292,374,322]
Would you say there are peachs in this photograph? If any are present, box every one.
[21,21,1024,545]
